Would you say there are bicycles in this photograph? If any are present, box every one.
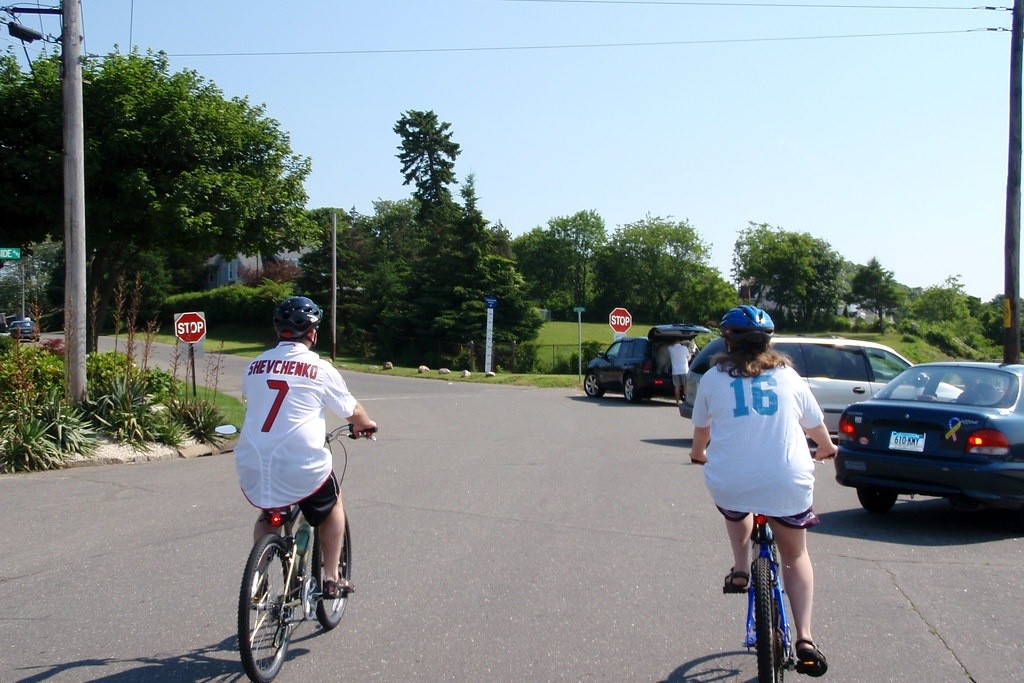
[691,450,834,683]
[237,422,378,683]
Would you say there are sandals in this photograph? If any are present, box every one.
[723,567,749,593]
[795,639,828,677]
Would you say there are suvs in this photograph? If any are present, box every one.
[679,334,965,458]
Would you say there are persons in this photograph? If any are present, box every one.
[668,338,692,406]
[688,304,838,677]
[234,296,378,612]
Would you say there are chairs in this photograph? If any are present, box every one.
[957,390,980,405]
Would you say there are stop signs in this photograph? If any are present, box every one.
[175,314,206,344]
[608,307,632,332]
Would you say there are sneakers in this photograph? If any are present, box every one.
[323,571,356,599]
[251,574,267,611]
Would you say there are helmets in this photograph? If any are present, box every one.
[273,296,323,338]
[720,305,775,338]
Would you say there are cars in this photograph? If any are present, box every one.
[835,362,1024,534]
[9,320,40,340]
[584,323,712,412]
